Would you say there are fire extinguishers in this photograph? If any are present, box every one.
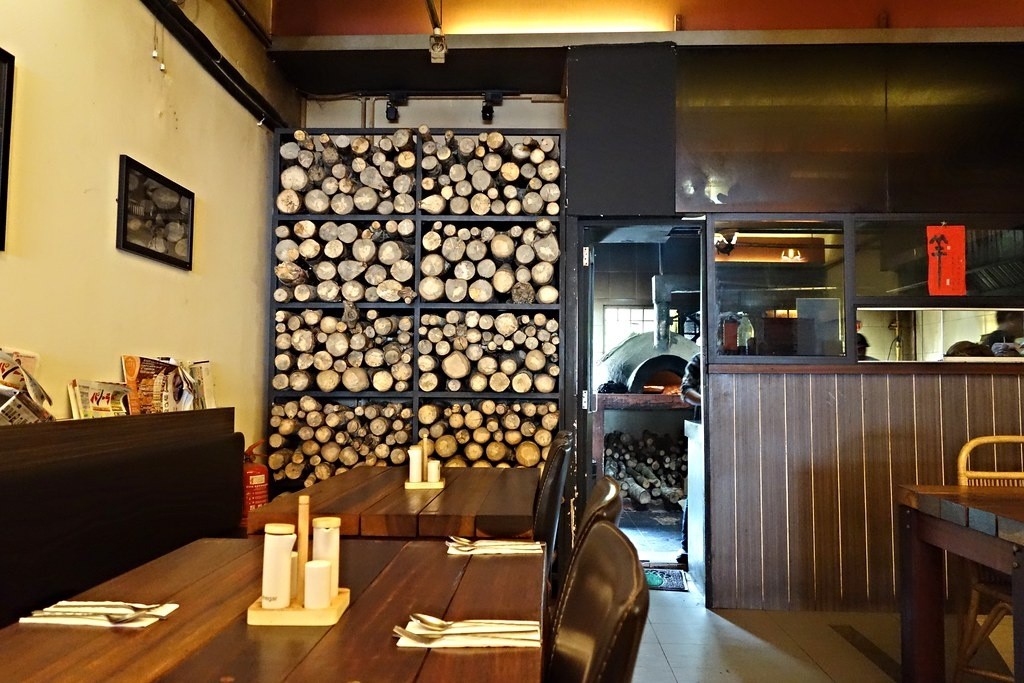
[242,439,270,535]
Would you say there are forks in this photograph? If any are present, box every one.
[54,596,174,614]
[393,625,542,644]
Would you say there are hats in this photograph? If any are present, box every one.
[855,332,869,348]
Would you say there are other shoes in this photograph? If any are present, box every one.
[676,552,687,564]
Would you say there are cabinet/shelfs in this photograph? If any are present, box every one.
[265,125,564,504]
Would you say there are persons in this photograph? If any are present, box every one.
[856,333,881,360]
[982,312,1024,346]
[678,315,758,561]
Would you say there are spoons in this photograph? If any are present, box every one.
[31,610,148,626]
[445,535,546,555]
[410,609,539,631]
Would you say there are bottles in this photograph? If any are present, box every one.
[721,314,739,353]
[406,444,441,484]
[262,513,341,612]
[736,312,756,357]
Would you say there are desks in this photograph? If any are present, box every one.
[892,482,1023,683]
[244,464,542,541]
[0,407,246,632]
[1,538,546,681]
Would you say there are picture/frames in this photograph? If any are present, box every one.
[0,43,16,252]
[116,155,195,273]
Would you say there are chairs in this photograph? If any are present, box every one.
[953,434,1024,683]
[536,429,573,594]
[564,475,621,582]
[550,520,650,683]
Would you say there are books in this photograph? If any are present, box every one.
[0,350,218,426]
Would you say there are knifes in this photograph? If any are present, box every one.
[45,608,169,621]
[390,628,538,638]
